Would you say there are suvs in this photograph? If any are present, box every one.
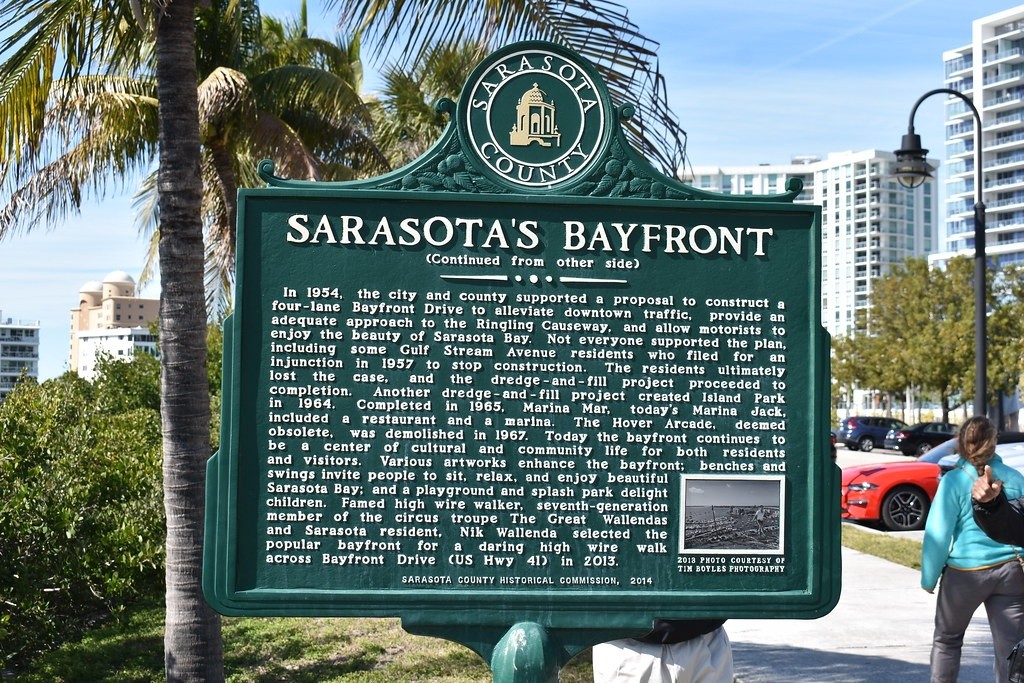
[841,416,909,452]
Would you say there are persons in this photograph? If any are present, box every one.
[592,619,733,683]
[970,464,1024,548]
[920,416,1024,683]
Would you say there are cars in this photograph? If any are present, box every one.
[892,421,959,459]
[842,430,1023,532]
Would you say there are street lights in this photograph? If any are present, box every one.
[886,87,988,417]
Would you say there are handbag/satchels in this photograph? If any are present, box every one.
[1006,639,1024,683]
